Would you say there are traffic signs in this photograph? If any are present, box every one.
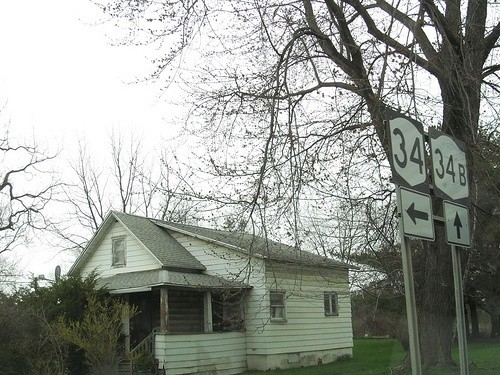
[386,106,470,247]
